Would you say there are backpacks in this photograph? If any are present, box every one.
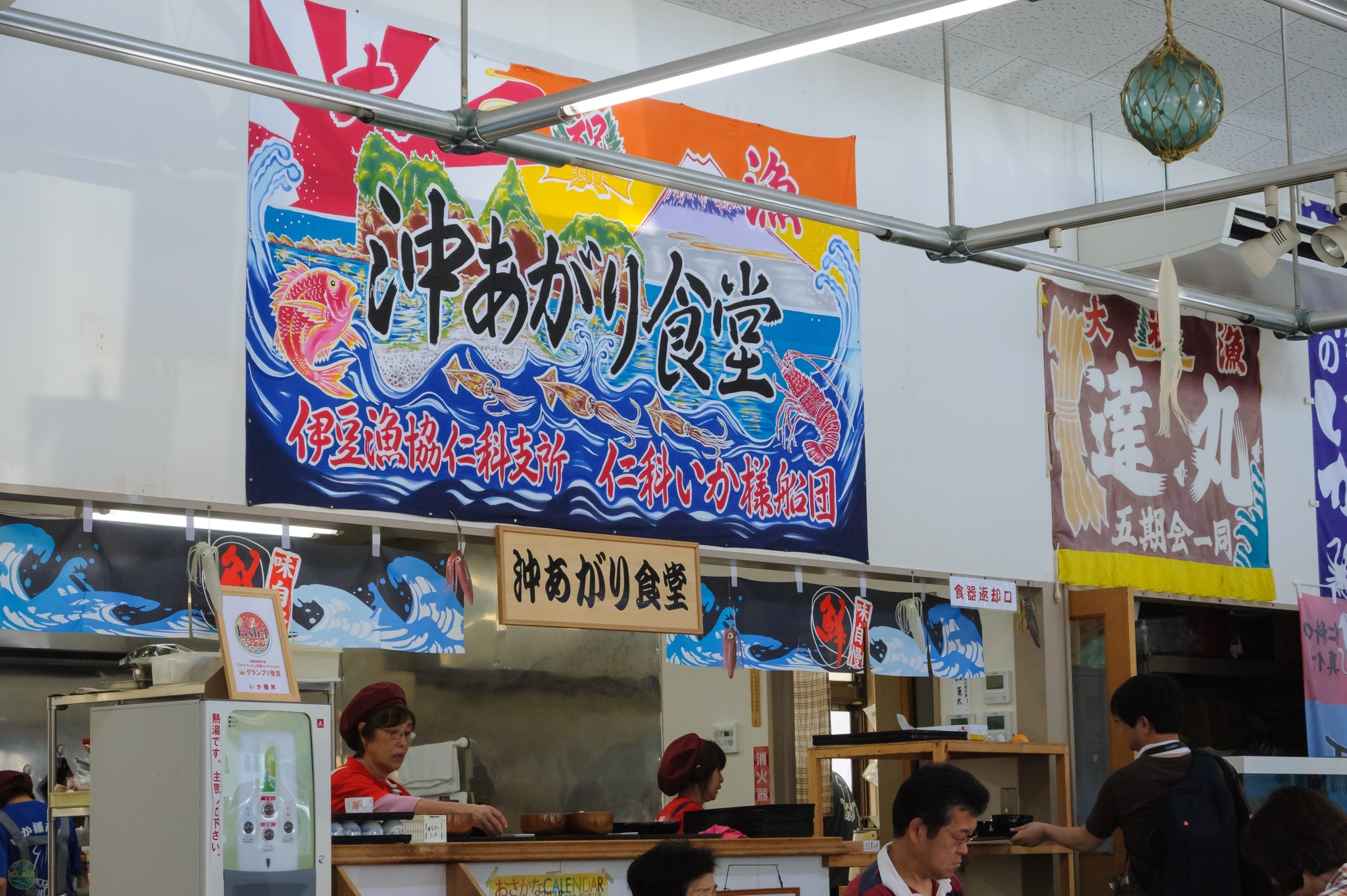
[1142,746,1244,896]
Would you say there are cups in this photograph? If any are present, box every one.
[995,734,1005,741]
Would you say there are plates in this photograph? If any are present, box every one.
[967,831,1003,837]
[101,680,152,690]
[611,821,682,834]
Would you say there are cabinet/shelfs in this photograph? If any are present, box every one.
[810,740,1076,896]
[49,681,338,896]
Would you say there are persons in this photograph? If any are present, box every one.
[1007,673,1347,896]
[627,837,718,896]
[0,771,83,896]
[654,733,726,834]
[842,763,990,896]
[331,682,507,837]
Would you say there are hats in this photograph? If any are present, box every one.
[657,733,707,797]
[339,681,407,752]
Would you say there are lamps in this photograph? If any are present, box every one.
[1309,171,1347,267]
[485,131,950,255]
[473,1,1033,145]
[1234,183,1304,279]
[969,247,1300,338]
[73,505,345,540]
[0,5,459,144]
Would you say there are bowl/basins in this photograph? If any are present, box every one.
[974,821,991,831]
[991,814,1034,835]
[520,814,569,835]
[569,812,614,835]
[345,797,373,813]
[290,643,344,679]
[151,651,223,685]
[331,819,361,836]
[357,818,405,835]
[130,667,151,680]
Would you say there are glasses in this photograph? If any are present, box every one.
[944,826,978,845]
[377,726,419,742]
